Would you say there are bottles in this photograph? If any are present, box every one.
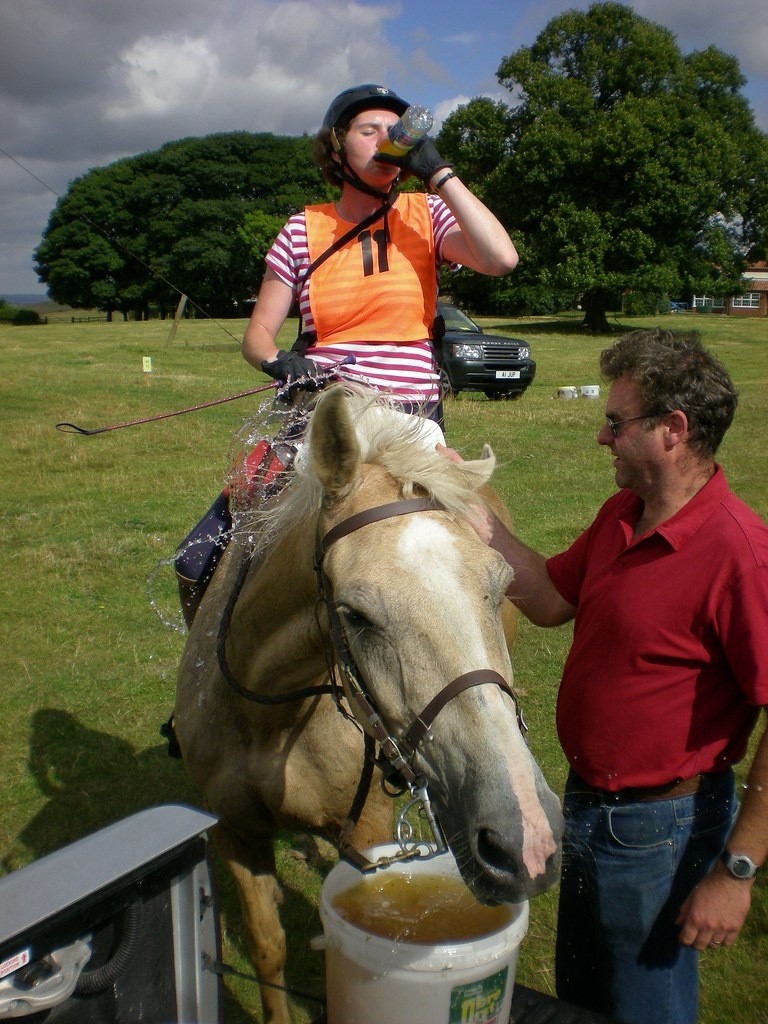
[376,104,433,168]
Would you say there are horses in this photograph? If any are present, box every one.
[169,378,567,1023]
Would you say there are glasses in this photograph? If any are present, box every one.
[605,412,671,438]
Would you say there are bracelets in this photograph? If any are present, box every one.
[434,172,457,196]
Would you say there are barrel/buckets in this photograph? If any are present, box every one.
[581,385,600,399]
[317,843,530,1024]
[557,386,576,398]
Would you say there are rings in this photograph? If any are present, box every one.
[710,940,723,947]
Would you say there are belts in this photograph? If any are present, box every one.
[568,769,733,808]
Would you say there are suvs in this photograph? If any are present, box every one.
[433,301,537,402]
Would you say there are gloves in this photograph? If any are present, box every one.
[371,125,454,189]
[261,351,331,393]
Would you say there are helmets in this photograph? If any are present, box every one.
[321,83,413,137]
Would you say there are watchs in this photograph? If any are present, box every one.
[719,847,761,882]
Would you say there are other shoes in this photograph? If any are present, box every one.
[169,726,182,759]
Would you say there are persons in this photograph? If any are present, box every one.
[163,84,522,756]
[433,327,768,1024]
